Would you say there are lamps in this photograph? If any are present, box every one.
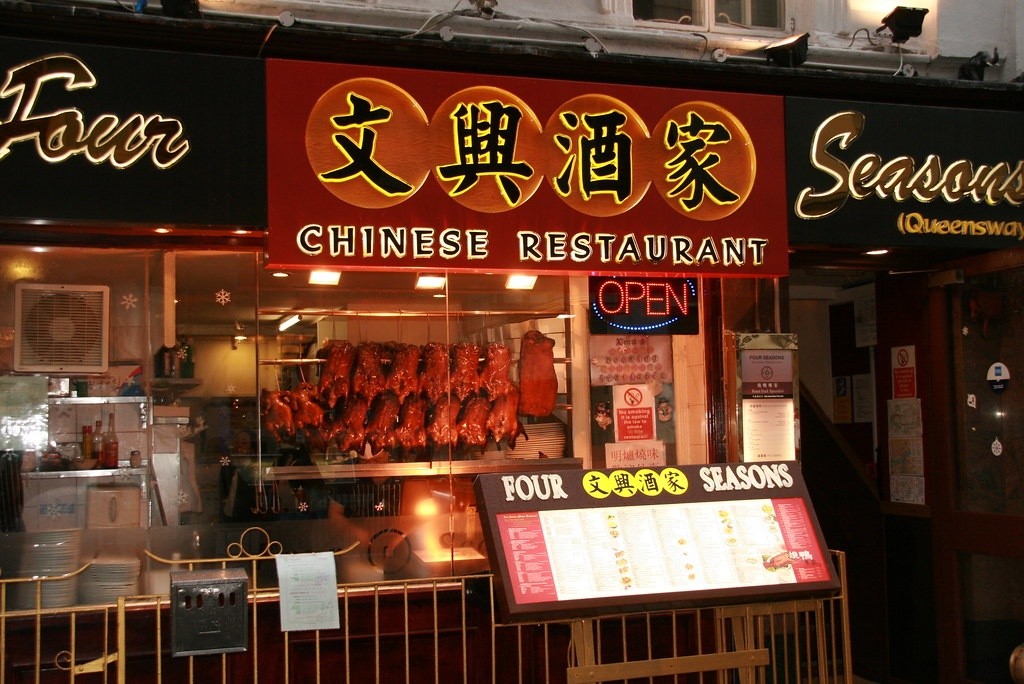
[765,32,809,68]
[878,7,930,44]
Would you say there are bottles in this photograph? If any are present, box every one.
[83,425,92,458]
[155,334,195,378]
[106,413,118,468]
[130,452,142,467]
[92,421,105,468]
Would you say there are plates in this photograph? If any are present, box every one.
[17,527,141,607]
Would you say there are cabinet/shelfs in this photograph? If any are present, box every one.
[18,393,154,530]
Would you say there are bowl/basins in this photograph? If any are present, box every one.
[72,459,97,469]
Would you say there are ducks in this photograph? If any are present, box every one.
[263,339,529,456]
